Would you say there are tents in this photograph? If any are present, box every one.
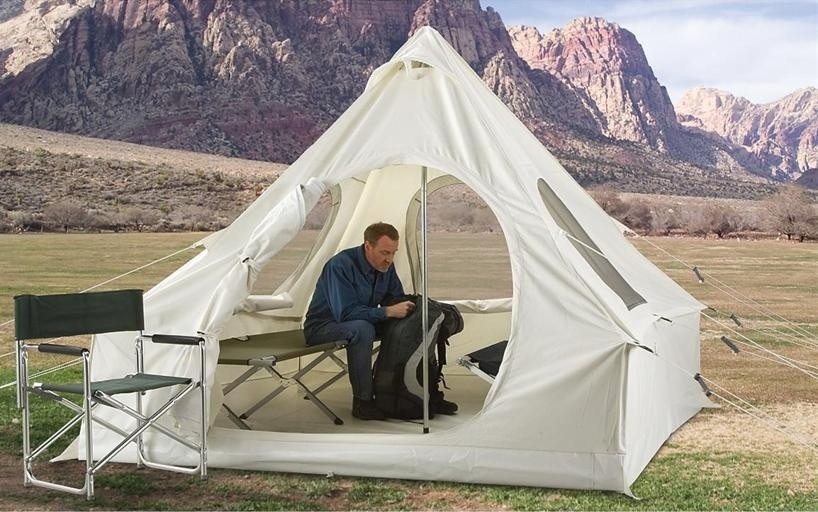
[49,26,721,501]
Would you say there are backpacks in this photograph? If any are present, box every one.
[372,294,464,420]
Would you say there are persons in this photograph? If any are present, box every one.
[303,222,458,420]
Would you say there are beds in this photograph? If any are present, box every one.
[457,339,507,385]
[218,329,381,429]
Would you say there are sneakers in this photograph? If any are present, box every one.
[352,397,385,420]
[429,391,458,414]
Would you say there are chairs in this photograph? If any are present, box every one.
[14,289,208,500]
